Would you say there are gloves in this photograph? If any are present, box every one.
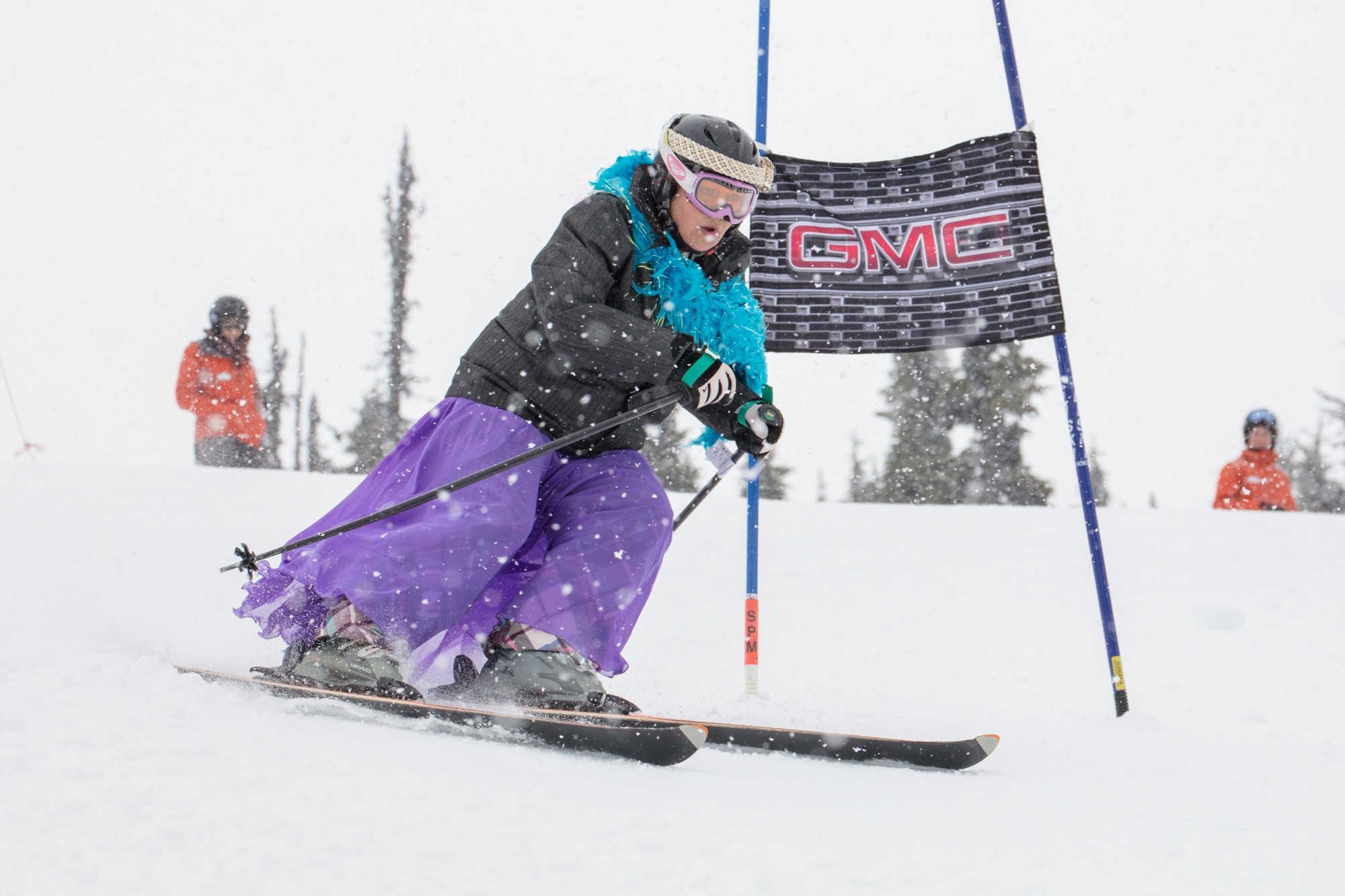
[669,344,736,411]
[729,396,776,457]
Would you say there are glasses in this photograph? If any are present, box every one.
[1248,410,1275,424]
[683,171,757,224]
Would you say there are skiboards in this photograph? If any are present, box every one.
[170,657,1003,773]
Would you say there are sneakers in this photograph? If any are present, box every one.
[480,649,625,715]
[291,634,423,716]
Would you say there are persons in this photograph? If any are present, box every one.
[230,112,784,711]
[1212,407,1297,511]
[175,296,272,468]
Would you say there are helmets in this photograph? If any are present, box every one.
[210,297,250,331]
[1244,410,1276,448]
[654,114,760,241]
